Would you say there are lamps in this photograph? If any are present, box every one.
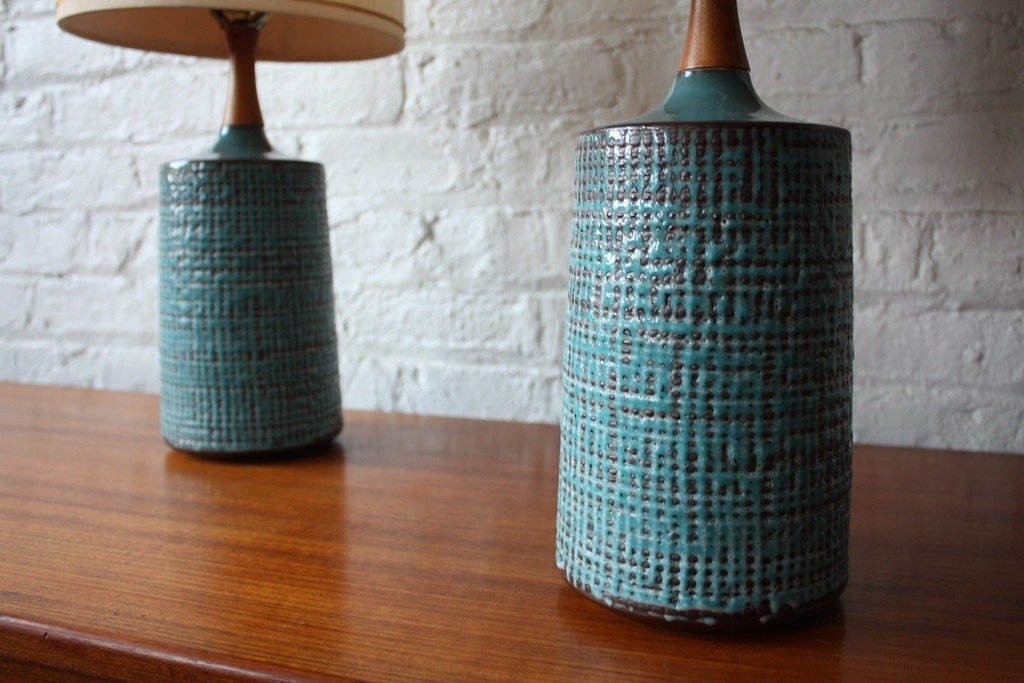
[54,1,407,457]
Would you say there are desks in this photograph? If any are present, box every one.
[1,378,1023,683]
